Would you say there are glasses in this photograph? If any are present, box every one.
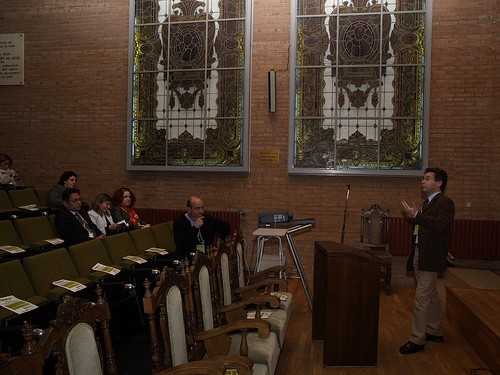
[65,197,82,202]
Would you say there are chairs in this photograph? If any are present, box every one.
[357,204,394,295]
[0,161,313,374]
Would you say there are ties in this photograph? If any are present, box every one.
[75,212,91,231]
[422,199,429,214]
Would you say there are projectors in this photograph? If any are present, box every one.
[257,209,292,223]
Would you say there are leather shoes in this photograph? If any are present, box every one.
[399,340,425,354]
[425,333,444,342]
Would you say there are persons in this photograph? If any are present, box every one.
[88,194,129,237]
[0,152,18,186]
[172,196,231,254]
[399,167,455,355]
[54,187,105,246]
[48,169,90,212]
[108,188,151,233]
